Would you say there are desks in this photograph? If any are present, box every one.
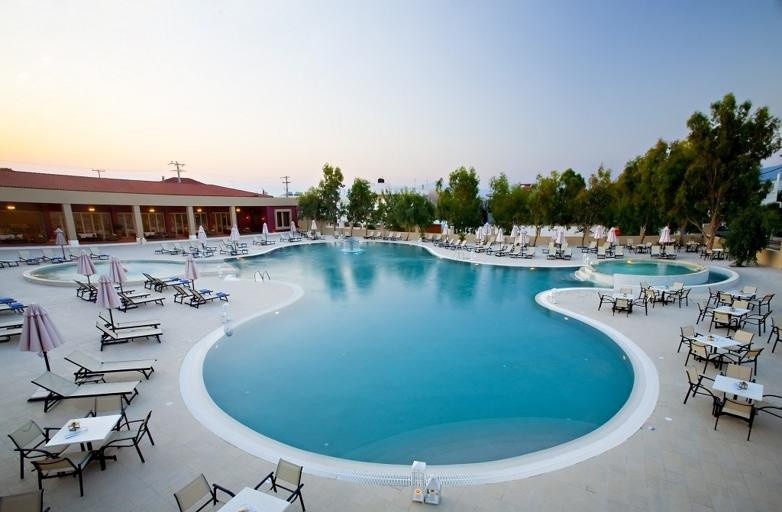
[217,486,292,512]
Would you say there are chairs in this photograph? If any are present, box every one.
[74,267,235,315]
[420,221,734,263]
[597,277,693,319]
[254,458,305,512]
[151,221,414,257]
[173,473,236,512]
[2,297,165,512]
[675,285,782,441]
[2,242,110,267]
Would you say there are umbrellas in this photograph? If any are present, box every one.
[659,226,671,248]
[551,224,616,258]
[109,256,128,294]
[476,222,529,257]
[198,226,208,249]
[185,254,198,290]
[54,227,67,259]
[443,223,450,242]
[262,222,268,241]
[230,223,240,250]
[290,220,297,237]
[19,304,64,371]
[77,249,96,285]
[310,220,317,239]
[96,272,121,332]
